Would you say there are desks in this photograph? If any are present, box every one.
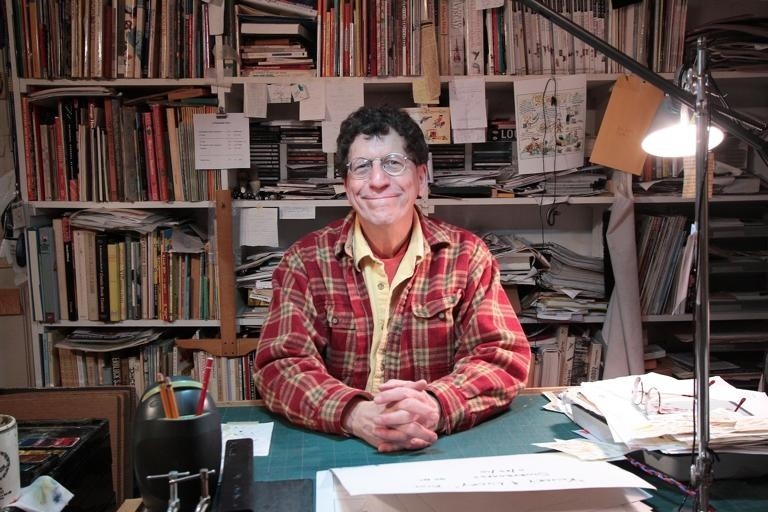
[213,386,768,512]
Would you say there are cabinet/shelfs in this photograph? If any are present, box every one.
[1,1,768,400]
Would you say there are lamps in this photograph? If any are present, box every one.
[641,36,728,511]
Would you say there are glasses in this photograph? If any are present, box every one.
[344,151,414,177]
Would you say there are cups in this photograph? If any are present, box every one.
[0,413,22,508]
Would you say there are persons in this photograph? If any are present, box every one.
[250,104,533,453]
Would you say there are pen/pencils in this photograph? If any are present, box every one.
[156,355,214,419]
